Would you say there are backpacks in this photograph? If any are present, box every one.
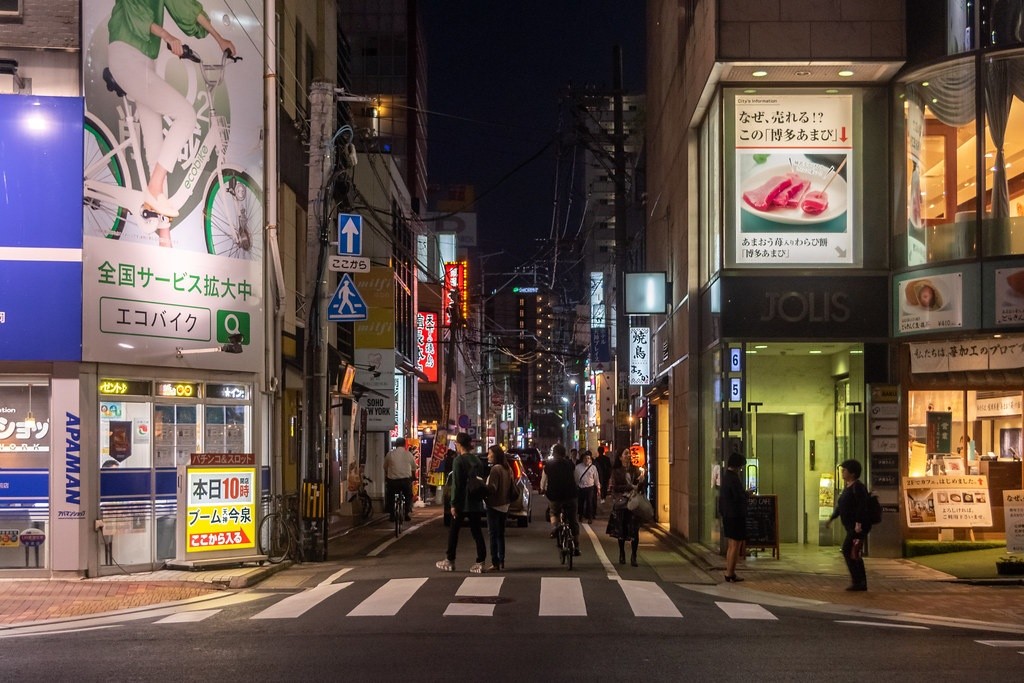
[853,483,883,525]
[459,454,490,501]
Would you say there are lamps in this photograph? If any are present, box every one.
[355,366,381,378]
[175,333,243,359]
[0,57,32,95]
[354,363,376,372]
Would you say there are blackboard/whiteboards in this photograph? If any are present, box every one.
[744,495,778,549]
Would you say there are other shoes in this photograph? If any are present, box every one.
[619,553,625,564]
[404,514,412,522]
[630,554,637,567]
[435,558,455,571]
[483,565,500,573]
[500,562,505,569]
[388,512,396,522]
[549,529,558,539]
[845,584,867,592]
[573,549,582,556]
[470,561,486,574]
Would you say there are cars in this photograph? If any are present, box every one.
[506,448,548,489]
[443,453,532,529]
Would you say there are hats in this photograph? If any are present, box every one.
[456,433,473,445]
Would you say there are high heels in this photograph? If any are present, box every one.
[725,573,744,583]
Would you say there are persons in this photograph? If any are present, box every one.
[540,444,582,555]
[718,453,754,581]
[576,455,600,524]
[384,436,417,521]
[570,447,613,503]
[826,460,877,592]
[108,0,236,248]
[607,447,645,566]
[959,435,978,459]
[436,432,487,573]
[481,445,512,573]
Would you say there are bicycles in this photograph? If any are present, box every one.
[257,491,302,563]
[84,42,264,261]
[551,504,580,571]
[394,490,409,538]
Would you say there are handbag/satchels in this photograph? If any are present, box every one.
[628,482,654,520]
[574,486,579,498]
[510,479,521,502]
[850,530,864,560]
[612,496,629,511]
